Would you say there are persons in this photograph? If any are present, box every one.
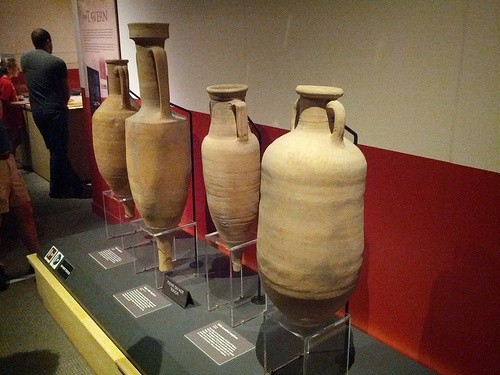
[0,56,25,167]
[0,102,42,279]
[21,28,92,199]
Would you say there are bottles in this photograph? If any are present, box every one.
[90,21,369,329]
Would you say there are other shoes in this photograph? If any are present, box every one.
[0,265,7,291]
[80,180,92,199]
